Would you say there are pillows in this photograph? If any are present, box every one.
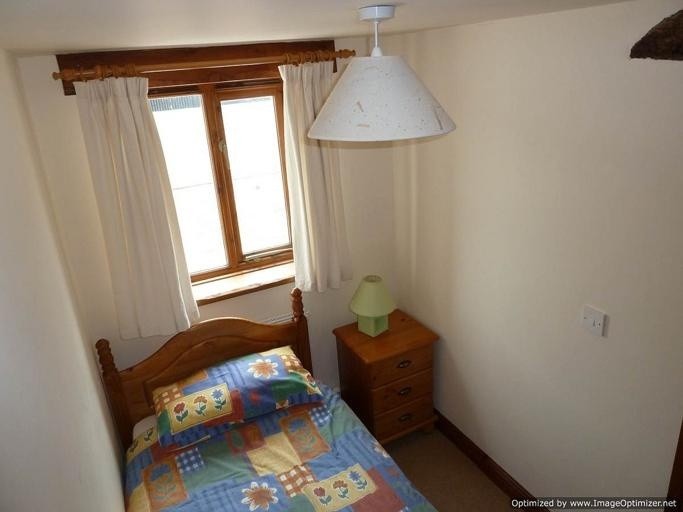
[150,343,328,456]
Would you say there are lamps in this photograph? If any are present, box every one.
[307,5,457,143]
[348,274,397,338]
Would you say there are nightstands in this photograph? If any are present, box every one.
[331,307,440,445]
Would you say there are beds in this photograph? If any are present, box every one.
[95,285,436,512]
